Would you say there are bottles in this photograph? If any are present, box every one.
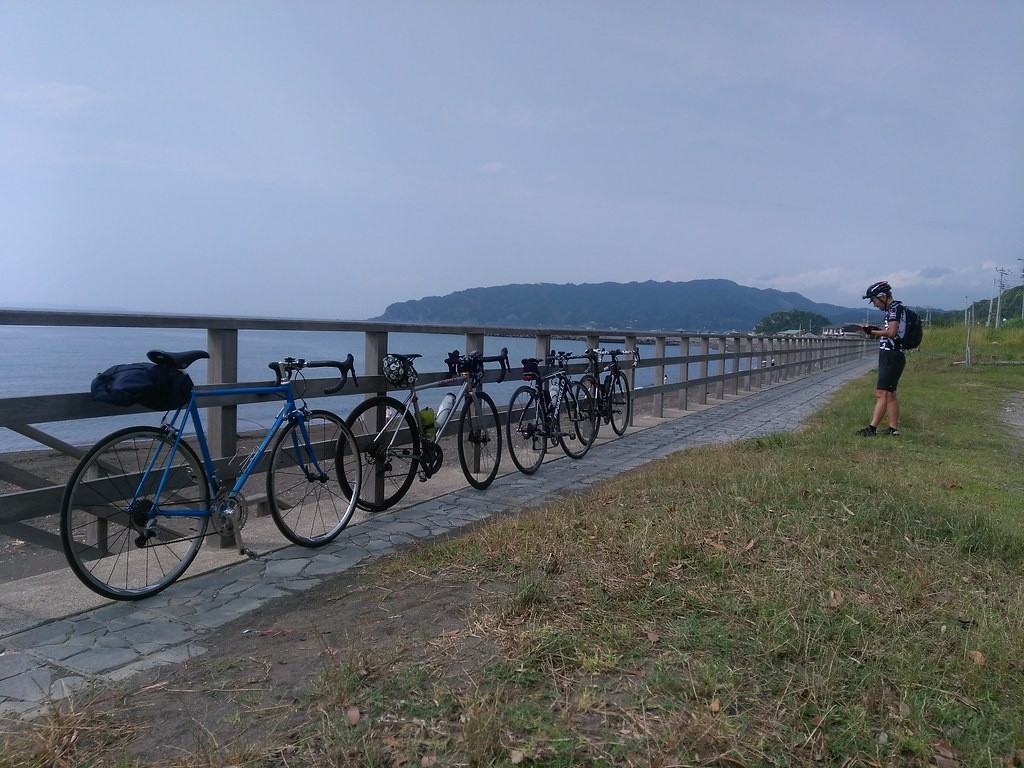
[604,375,611,392]
[663,375,668,384]
[549,382,559,407]
[421,407,436,440]
[434,392,456,428]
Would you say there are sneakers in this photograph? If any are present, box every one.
[857,426,877,437]
[883,427,899,435]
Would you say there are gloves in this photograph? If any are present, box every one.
[862,324,880,335]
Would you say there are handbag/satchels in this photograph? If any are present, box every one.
[89,362,193,411]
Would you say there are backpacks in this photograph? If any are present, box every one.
[888,304,923,351]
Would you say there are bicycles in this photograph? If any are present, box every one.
[573,346,640,446]
[335,346,512,513]
[59,347,363,601]
[505,348,596,474]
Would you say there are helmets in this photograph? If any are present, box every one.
[383,355,418,388]
[862,281,891,298]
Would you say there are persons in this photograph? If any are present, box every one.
[855,280,907,437]
